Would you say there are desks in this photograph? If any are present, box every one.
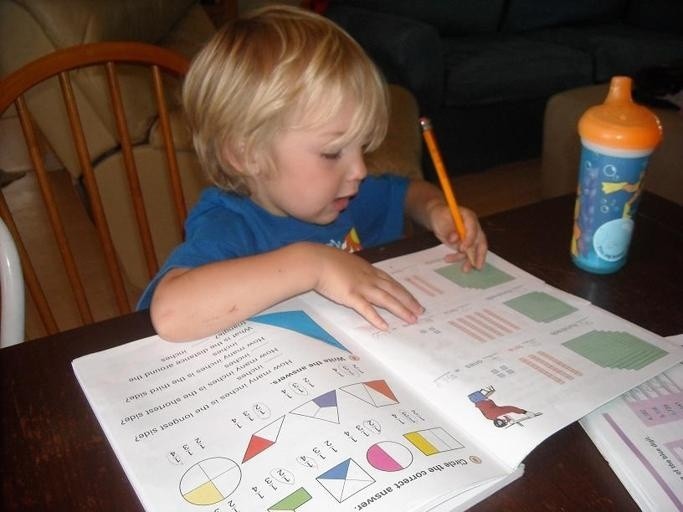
[0,180,682,512]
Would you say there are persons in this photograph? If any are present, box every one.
[133,3,491,344]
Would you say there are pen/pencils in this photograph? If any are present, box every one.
[418,117,477,270]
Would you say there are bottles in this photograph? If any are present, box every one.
[568,74,664,276]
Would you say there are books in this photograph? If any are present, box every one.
[578,331,681,512]
[69,242,683,512]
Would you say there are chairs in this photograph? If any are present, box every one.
[0,43,213,342]
